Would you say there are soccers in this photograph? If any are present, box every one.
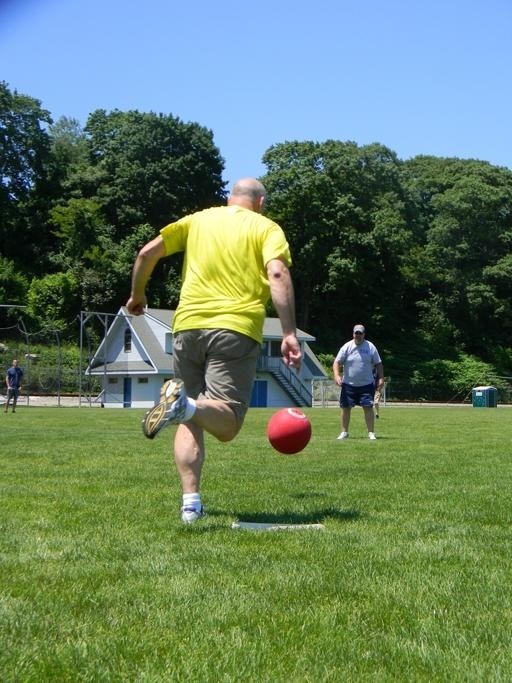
[267,408,311,453]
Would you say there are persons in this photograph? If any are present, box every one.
[373,380,383,419]
[3,358,24,414]
[126,176,302,526]
[332,323,385,441]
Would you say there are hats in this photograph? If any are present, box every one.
[354,324,365,335]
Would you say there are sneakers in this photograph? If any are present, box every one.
[368,431,376,441]
[180,505,207,523]
[141,377,187,439]
[336,431,349,440]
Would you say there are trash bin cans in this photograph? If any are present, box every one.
[472,386,497,407]
